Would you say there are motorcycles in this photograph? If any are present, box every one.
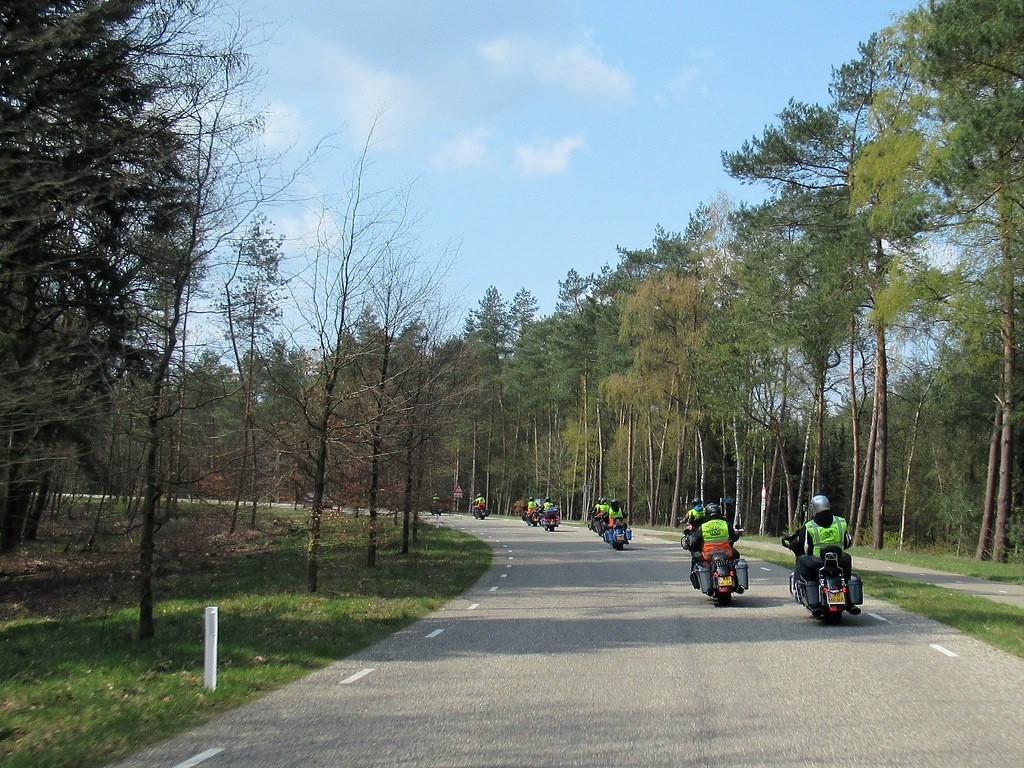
[536,505,560,531]
[522,504,543,526]
[677,517,695,550]
[430,501,443,516]
[603,511,632,550]
[781,535,865,625]
[588,507,609,536]
[683,529,749,607]
[472,502,490,520]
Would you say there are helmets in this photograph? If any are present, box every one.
[598,498,602,504]
[477,493,481,497]
[704,503,721,517]
[811,495,830,517]
[601,498,607,504]
[610,500,618,506]
[529,496,534,501]
[693,498,702,506]
[546,496,551,502]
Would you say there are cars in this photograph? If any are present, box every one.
[304,493,334,510]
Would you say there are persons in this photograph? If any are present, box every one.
[433,493,440,504]
[684,503,740,559]
[793,495,852,582]
[595,497,610,520]
[540,496,554,513]
[523,496,538,512]
[472,491,486,515]
[682,497,706,530]
[589,496,602,515]
[598,499,628,534]
